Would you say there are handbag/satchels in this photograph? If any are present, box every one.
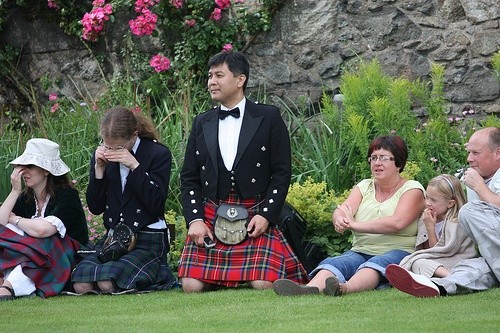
[214,204,247,243]
[98,224,136,262]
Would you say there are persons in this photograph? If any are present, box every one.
[68,106,181,294]
[272,134,426,298]
[0,137,90,301]
[384,126,500,298]
[177,49,309,295]
[398,173,477,278]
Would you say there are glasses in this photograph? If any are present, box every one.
[100,139,127,152]
[368,156,395,161]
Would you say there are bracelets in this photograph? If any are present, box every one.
[15,216,22,228]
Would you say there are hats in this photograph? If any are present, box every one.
[10,138,70,175]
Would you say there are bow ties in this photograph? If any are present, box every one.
[219,108,240,120]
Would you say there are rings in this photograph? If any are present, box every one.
[260,229,266,234]
[338,228,340,231]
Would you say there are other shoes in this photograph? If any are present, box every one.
[273,280,319,296]
[0,286,16,300]
[323,277,340,296]
[385,265,440,297]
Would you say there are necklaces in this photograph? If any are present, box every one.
[379,181,400,203]
